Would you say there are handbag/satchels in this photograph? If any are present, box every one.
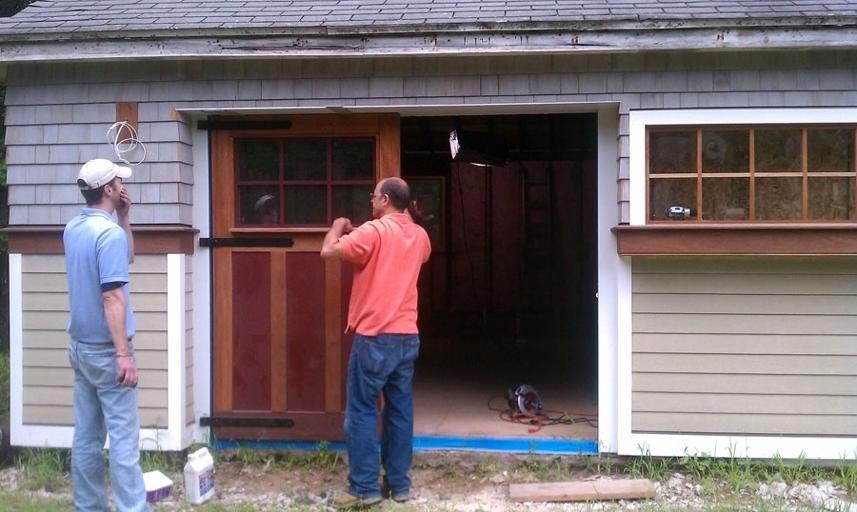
[115,353,132,359]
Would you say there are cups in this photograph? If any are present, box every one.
[369,192,384,197]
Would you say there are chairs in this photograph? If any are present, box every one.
[328,491,383,510]
[381,489,408,504]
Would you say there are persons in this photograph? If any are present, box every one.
[253,192,277,225]
[63,158,148,512]
[320,176,432,509]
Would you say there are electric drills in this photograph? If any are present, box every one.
[665,206,697,219]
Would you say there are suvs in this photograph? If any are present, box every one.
[181,446,217,505]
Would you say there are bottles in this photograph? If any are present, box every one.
[75,159,133,190]
